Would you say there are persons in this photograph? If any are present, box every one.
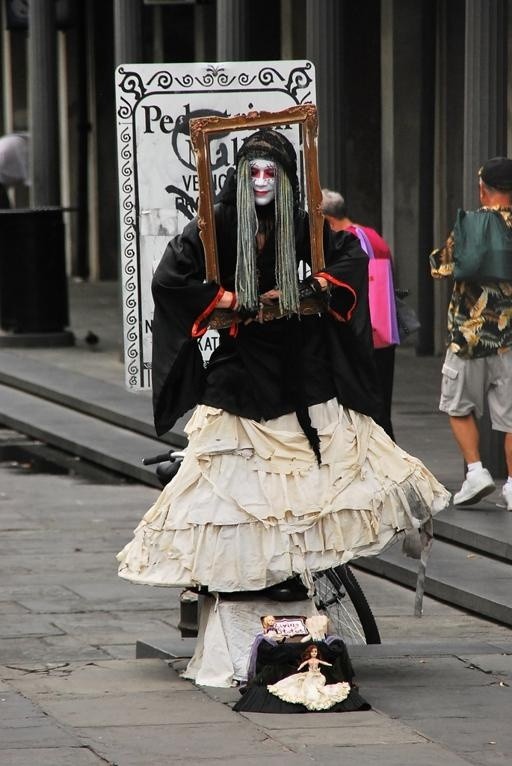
[318,186,399,446]
[264,615,287,644]
[147,127,451,601]
[266,644,350,712]
[427,155,512,513]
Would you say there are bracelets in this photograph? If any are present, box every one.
[227,291,240,311]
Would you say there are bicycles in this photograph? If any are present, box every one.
[142,450,381,645]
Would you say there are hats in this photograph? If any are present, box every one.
[233,129,298,187]
[477,156,512,194]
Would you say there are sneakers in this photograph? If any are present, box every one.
[501,484,512,510]
[453,469,496,508]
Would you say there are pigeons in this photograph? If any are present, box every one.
[84,328,101,351]
